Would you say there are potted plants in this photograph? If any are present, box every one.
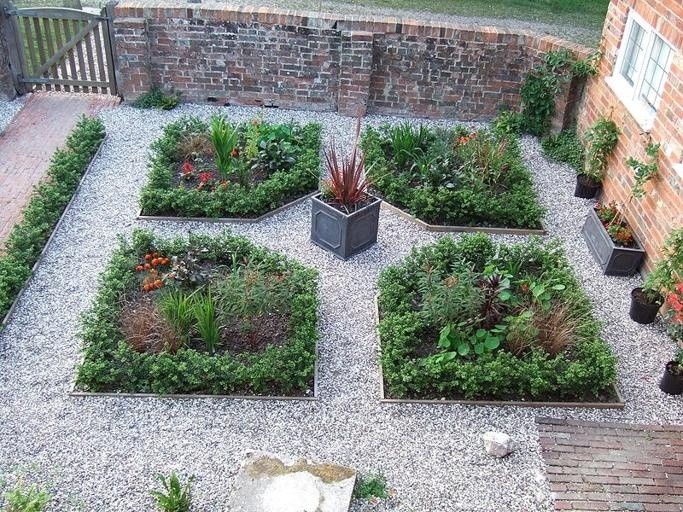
[574,119,618,198]
[582,131,661,278]
[628,227,682,325]
[307,115,396,261]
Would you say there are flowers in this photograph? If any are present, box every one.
[667,283,682,343]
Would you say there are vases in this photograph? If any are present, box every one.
[659,362,682,394]
